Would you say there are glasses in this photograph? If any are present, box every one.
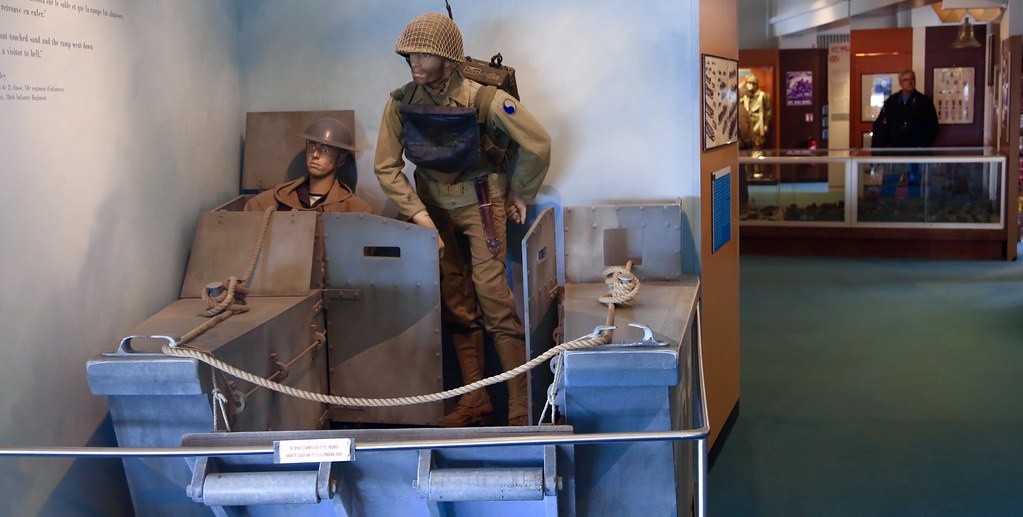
[901,77,914,82]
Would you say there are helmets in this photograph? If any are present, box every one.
[395,12,465,65]
[296,117,361,153]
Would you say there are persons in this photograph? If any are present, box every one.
[870,68,942,196]
[739,75,771,180]
[373,10,553,425]
[238,117,375,217]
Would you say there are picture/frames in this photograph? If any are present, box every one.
[739,67,774,150]
[933,68,975,124]
[785,72,813,106]
[860,73,903,121]
[702,54,738,151]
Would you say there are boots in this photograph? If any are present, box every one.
[443,328,494,426]
[493,339,528,426]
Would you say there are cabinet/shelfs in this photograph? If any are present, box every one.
[739,147,1015,262]
[1001,35,1023,246]
